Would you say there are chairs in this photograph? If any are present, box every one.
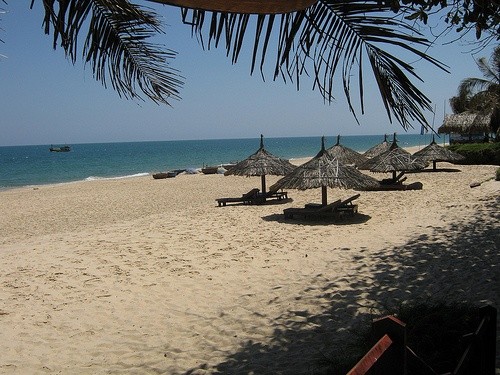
[376,171,407,190]
[284,192,362,221]
[215,184,287,207]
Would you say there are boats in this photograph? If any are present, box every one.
[49,144,72,152]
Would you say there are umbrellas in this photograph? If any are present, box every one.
[409,133,465,171]
[225,134,299,194]
[371,132,422,181]
[362,133,394,161]
[437,107,499,142]
[270,136,380,207]
[322,133,370,170]
